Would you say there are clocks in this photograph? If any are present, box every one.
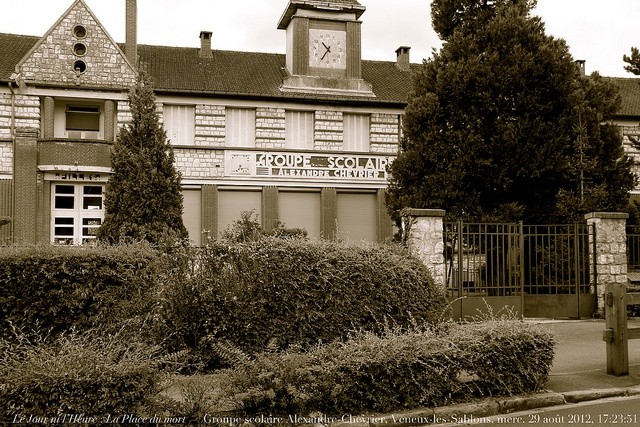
[307,29,347,69]
